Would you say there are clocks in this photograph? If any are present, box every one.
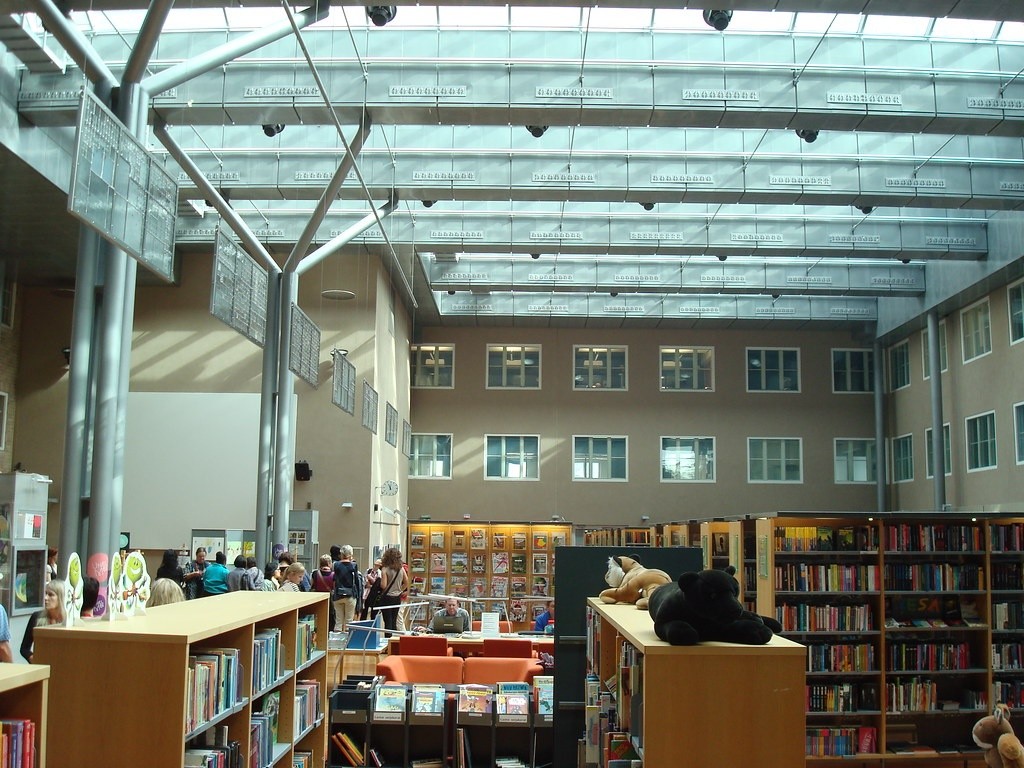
[382,481,398,497]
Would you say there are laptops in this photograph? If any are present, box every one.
[433,616,463,634]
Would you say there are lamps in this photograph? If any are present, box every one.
[365,3,396,26]
[420,198,911,300]
[261,122,285,136]
[702,9,733,30]
[797,130,820,143]
[524,125,550,138]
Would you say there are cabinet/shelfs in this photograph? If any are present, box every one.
[0,658,51,767]
[585,598,807,768]
[756,516,1024,768]
[33,590,330,768]
[581,519,756,612]
[407,522,572,623]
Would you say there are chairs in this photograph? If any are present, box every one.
[376,655,541,691]
[482,639,538,659]
[397,634,454,658]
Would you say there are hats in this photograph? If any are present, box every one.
[373,559,382,566]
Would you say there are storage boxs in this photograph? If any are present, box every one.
[349,620,380,649]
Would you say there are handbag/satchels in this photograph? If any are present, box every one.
[374,593,383,612]
[331,589,334,607]
[352,584,358,599]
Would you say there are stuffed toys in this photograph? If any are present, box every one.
[598,554,672,610]
[649,566,782,645]
[972,703,1024,768]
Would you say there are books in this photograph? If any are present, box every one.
[186,615,322,768]
[774,523,1024,755]
[578,605,644,768]
[328,629,349,650]
[0,719,35,768]
[409,528,566,621]
[586,533,757,614]
[331,675,553,768]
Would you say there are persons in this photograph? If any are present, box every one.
[535,601,555,635]
[414,594,469,634]
[0,603,13,663]
[146,544,410,638]
[20,544,100,664]
